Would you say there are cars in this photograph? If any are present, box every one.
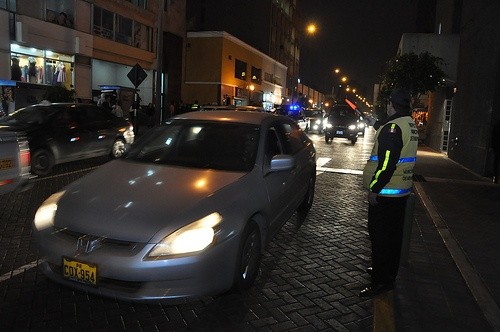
[0,103,135,194]
[33,110,316,307]
[277,104,365,145]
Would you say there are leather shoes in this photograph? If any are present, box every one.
[358,286,379,298]
[367,267,373,274]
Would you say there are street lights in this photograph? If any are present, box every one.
[334,68,373,108]
[291,24,316,104]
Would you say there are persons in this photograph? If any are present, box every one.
[11,58,22,80]
[358,88,419,299]
[97,91,202,133]
[51,12,67,27]
